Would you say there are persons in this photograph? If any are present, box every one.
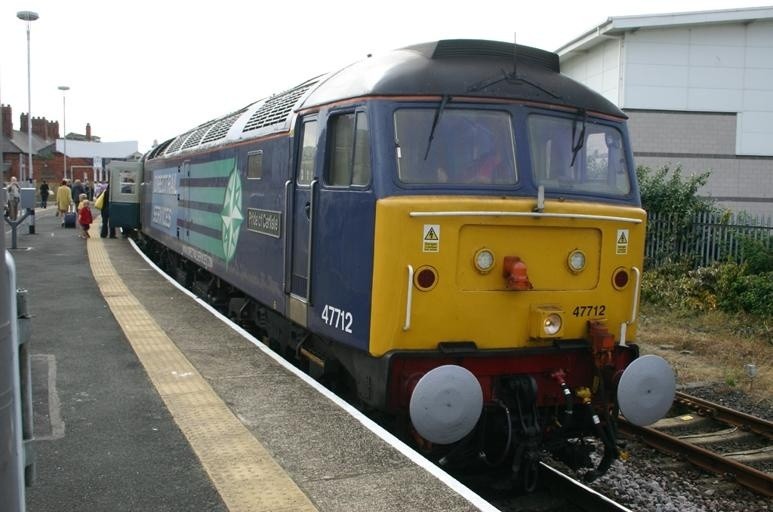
[6,177,21,220]
[54,177,136,239]
[39,180,50,208]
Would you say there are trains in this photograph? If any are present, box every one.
[108,39,677,497]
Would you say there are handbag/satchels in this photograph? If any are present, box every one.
[94,188,107,209]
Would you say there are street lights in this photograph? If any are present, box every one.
[16,11,39,188]
[58,86,69,179]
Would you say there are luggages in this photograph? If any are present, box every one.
[64,202,77,228]
